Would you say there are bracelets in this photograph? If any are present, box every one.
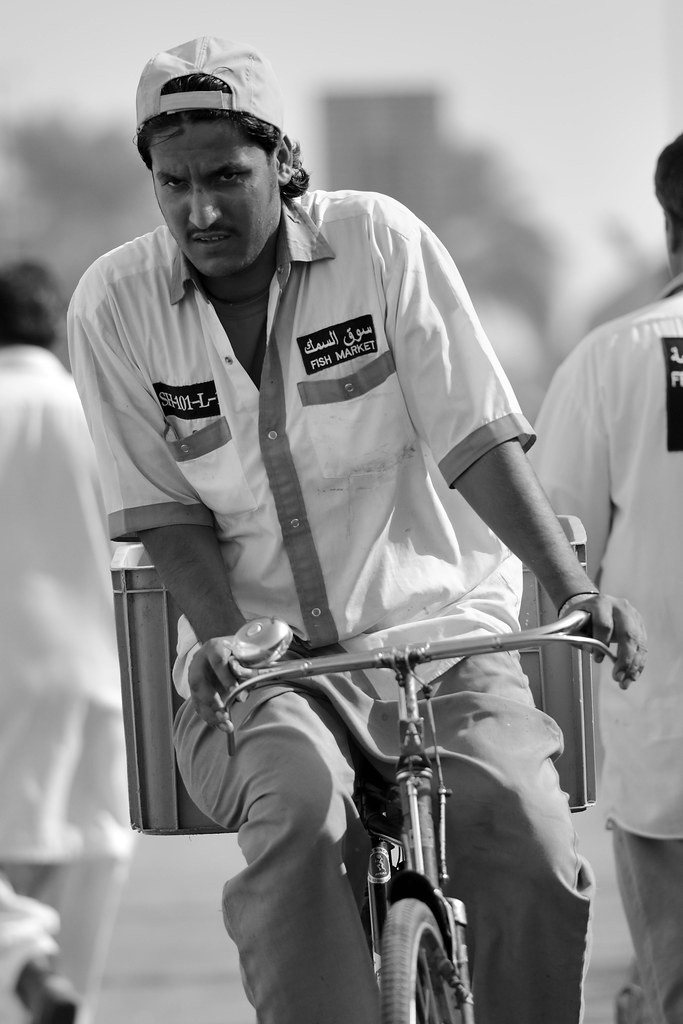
[556,589,601,620]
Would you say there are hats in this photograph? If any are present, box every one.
[134,35,283,134]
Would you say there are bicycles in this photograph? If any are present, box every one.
[112,517,637,1024]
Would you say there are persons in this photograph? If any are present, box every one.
[523,131,683,1023]
[1,256,144,1023]
[63,35,652,1024]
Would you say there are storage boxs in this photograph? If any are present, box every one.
[107,515,607,839]
